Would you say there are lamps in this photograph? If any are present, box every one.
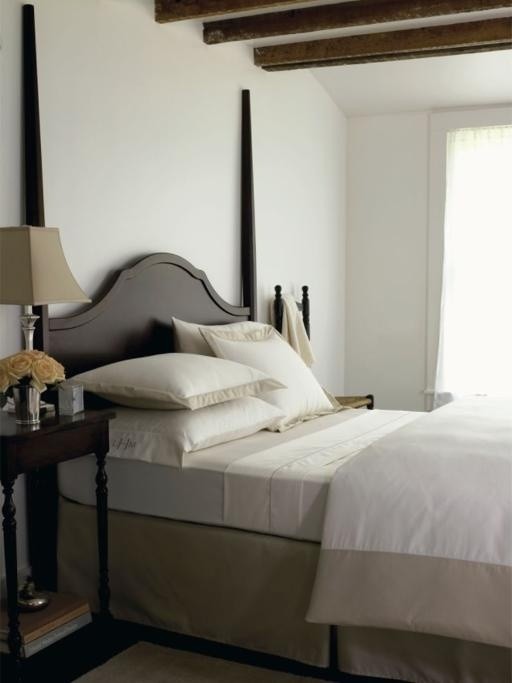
[0,225,95,416]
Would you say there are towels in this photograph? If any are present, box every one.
[268,293,318,369]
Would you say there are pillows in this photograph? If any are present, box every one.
[89,396,288,471]
[171,318,271,357]
[60,353,288,411]
[199,327,343,432]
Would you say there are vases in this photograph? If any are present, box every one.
[12,386,42,425]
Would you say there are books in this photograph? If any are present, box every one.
[0,592,94,658]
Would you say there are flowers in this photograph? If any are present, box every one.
[0,351,66,407]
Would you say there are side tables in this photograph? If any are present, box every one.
[0,404,121,683]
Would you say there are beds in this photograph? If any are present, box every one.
[29,246,511,679]
